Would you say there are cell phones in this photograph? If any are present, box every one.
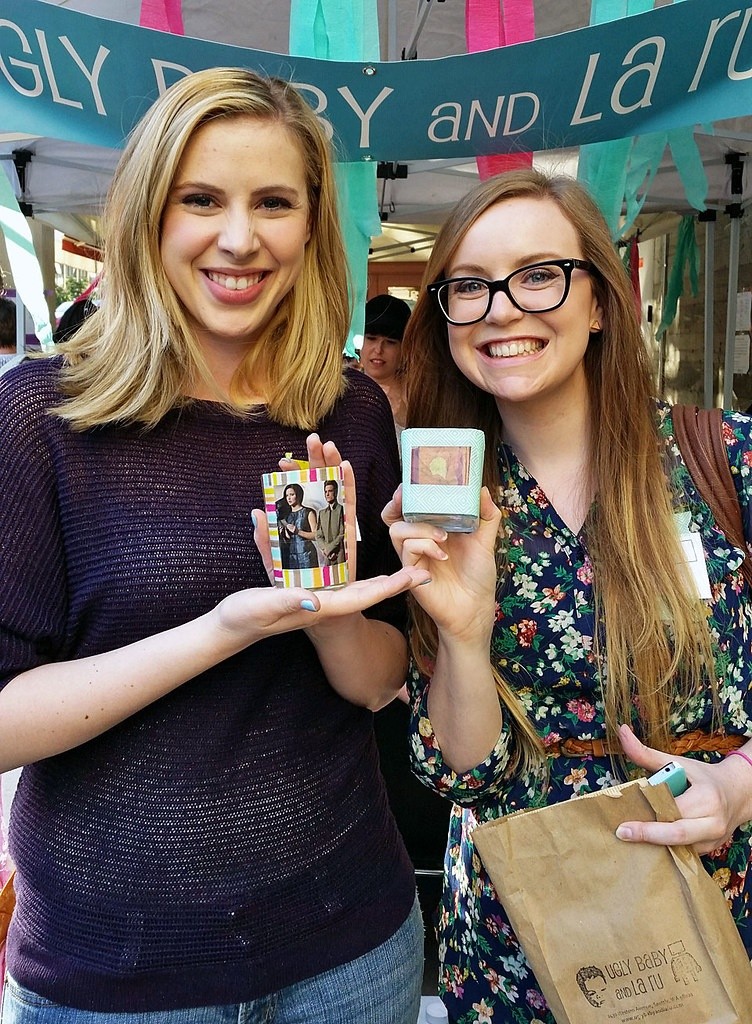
[648,761,688,796]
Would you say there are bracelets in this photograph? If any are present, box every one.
[726,751,752,764]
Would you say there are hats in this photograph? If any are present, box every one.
[365,295,412,341]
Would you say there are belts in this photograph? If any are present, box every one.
[547,734,748,757]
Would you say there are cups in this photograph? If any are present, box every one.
[401,428,485,533]
[262,465,352,588]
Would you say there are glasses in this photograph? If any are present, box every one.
[427,258,601,326]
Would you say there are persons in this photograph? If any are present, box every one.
[0,296,98,369]
[276,484,318,569]
[317,480,346,566]
[0,65,432,1024]
[381,170,752,1024]
[360,294,412,454]
